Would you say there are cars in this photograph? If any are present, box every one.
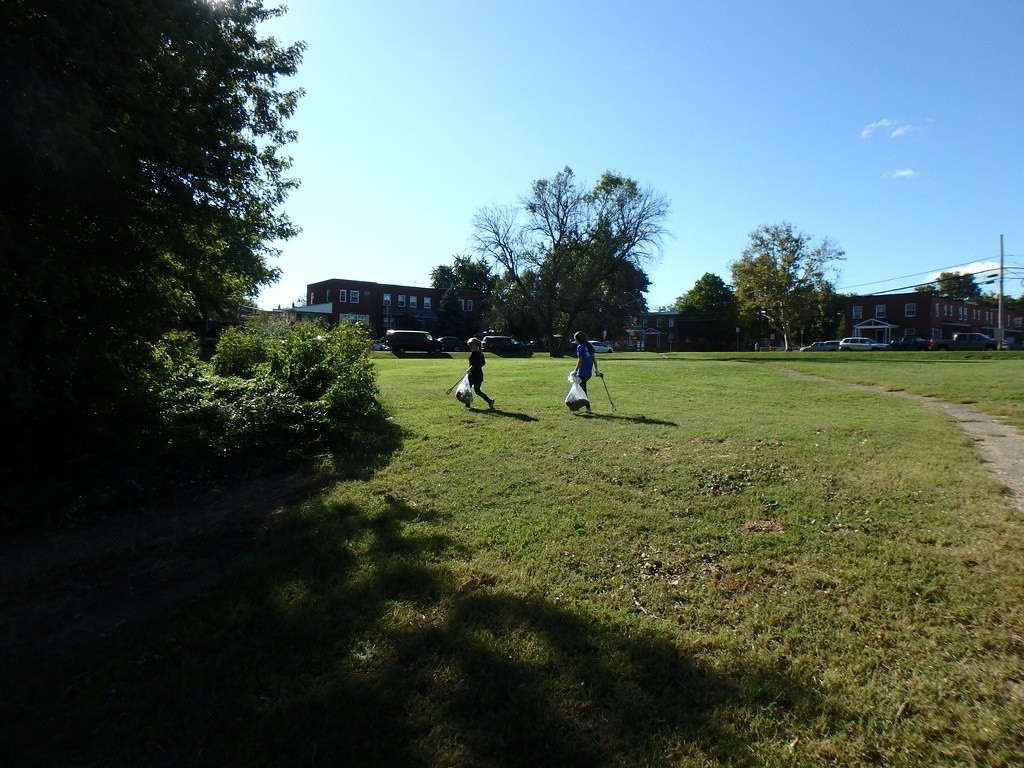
[588,340,613,353]
[888,334,929,352]
[436,336,464,352]
[800,340,840,352]
[370,343,388,351]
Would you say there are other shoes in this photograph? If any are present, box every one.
[573,411,581,414]
[585,410,592,414]
[465,405,470,409]
[489,399,494,409]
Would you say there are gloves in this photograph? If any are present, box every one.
[571,369,578,375]
[596,369,600,376]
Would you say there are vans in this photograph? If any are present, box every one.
[384,330,445,358]
[482,335,531,353]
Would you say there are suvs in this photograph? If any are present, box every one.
[839,336,891,351]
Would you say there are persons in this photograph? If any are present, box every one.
[574,332,600,414]
[465,338,494,410]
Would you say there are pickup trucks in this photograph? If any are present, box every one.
[929,332,1009,351]
